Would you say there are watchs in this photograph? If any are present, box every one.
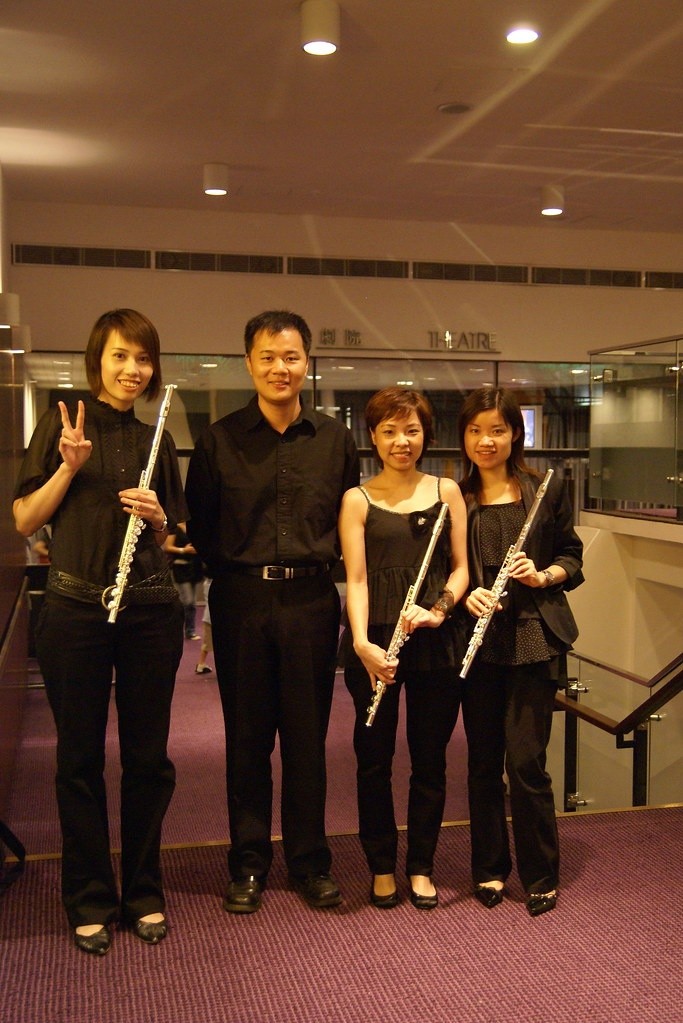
[148,514,167,532]
[540,570,554,589]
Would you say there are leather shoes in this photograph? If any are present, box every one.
[223,875,264,912]
[475,882,504,908]
[409,879,438,909]
[371,873,397,907]
[293,872,342,908]
[526,892,557,915]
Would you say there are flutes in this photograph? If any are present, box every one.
[363,501,449,724]
[459,469,555,677]
[107,383,179,624]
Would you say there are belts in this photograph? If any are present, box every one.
[46,567,179,612]
[220,563,330,580]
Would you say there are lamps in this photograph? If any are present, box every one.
[506,16,540,44]
[540,184,564,216]
[11,325,31,353]
[0,292,20,328]
[299,0,341,55]
[202,163,227,196]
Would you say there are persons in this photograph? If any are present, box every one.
[336,386,469,908]
[184,309,360,913]
[161,520,216,673]
[11,307,186,956]
[33,534,50,563]
[451,387,584,915]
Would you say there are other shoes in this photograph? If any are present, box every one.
[73,923,112,955]
[135,913,167,943]
